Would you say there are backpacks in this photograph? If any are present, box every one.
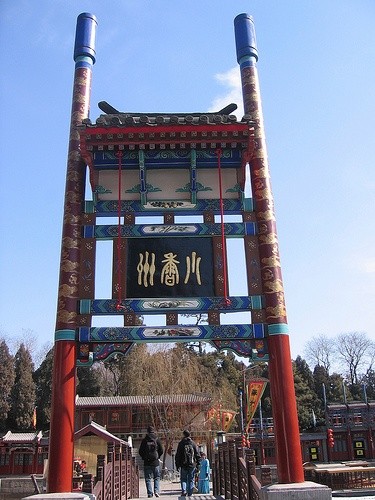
[144,437,159,462]
[181,440,195,465]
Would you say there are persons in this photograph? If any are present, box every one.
[198,453,210,494]
[138,425,164,498]
[175,430,200,496]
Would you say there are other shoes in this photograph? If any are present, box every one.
[181,491,187,496]
[154,492,159,498]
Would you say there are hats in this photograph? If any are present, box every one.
[147,426,154,433]
